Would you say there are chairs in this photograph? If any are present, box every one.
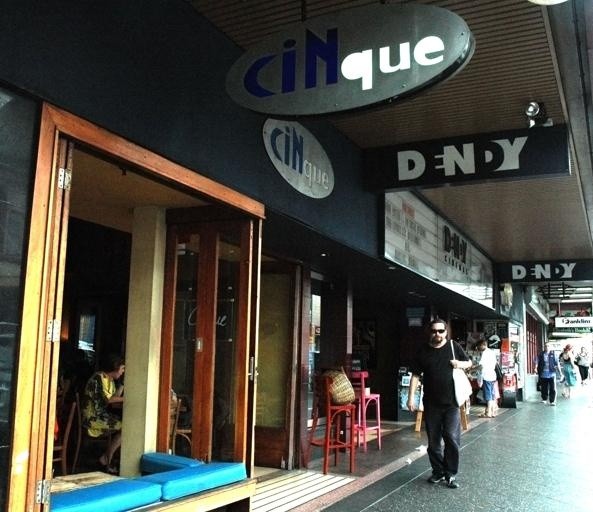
[307,370,382,476]
[52,372,191,476]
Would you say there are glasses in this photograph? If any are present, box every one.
[430,328,446,334]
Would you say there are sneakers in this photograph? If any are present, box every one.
[444,476,460,488]
[427,472,444,484]
[539,399,546,404]
[547,401,556,406]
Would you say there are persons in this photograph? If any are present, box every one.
[559,343,576,399]
[405,317,473,488]
[575,346,590,385]
[79,354,124,476]
[532,341,565,406]
[472,338,497,418]
[533,348,541,392]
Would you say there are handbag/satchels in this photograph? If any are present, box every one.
[475,369,484,388]
[450,367,473,407]
[495,362,503,380]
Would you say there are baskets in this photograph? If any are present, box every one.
[319,369,356,405]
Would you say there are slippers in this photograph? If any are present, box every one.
[477,413,496,418]
